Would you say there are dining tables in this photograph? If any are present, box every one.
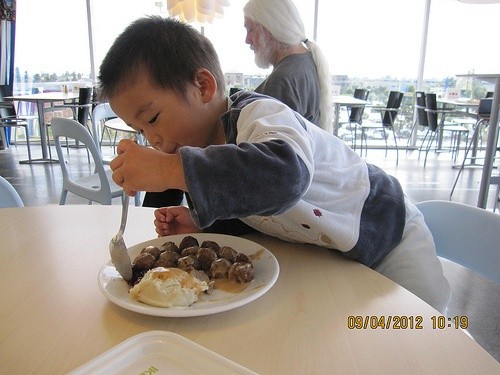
[0,201,500,375]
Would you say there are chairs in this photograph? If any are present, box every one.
[91,101,120,204]
[0,176,25,208]
[420,92,469,168]
[412,199,500,303]
[333,87,371,156]
[406,90,460,160]
[47,86,92,162]
[357,90,406,164]
[466,98,493,160]
[50,116,136,205]
[90,86,109,114]
[0,85,34,161]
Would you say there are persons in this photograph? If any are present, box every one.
[96,12,451,317]
[142,0,333,208]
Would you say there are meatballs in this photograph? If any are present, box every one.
[129,235,253,285]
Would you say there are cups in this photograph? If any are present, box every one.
[62,84,69,94]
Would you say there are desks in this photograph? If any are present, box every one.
[331,94,368,137]
[7,89,81,164]
[104,117,140,134]
[437,98,497,170]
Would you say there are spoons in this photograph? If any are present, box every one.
[109,191,132,280]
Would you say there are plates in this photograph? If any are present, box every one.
[66,331,259,375]
[97,233,279,317]
